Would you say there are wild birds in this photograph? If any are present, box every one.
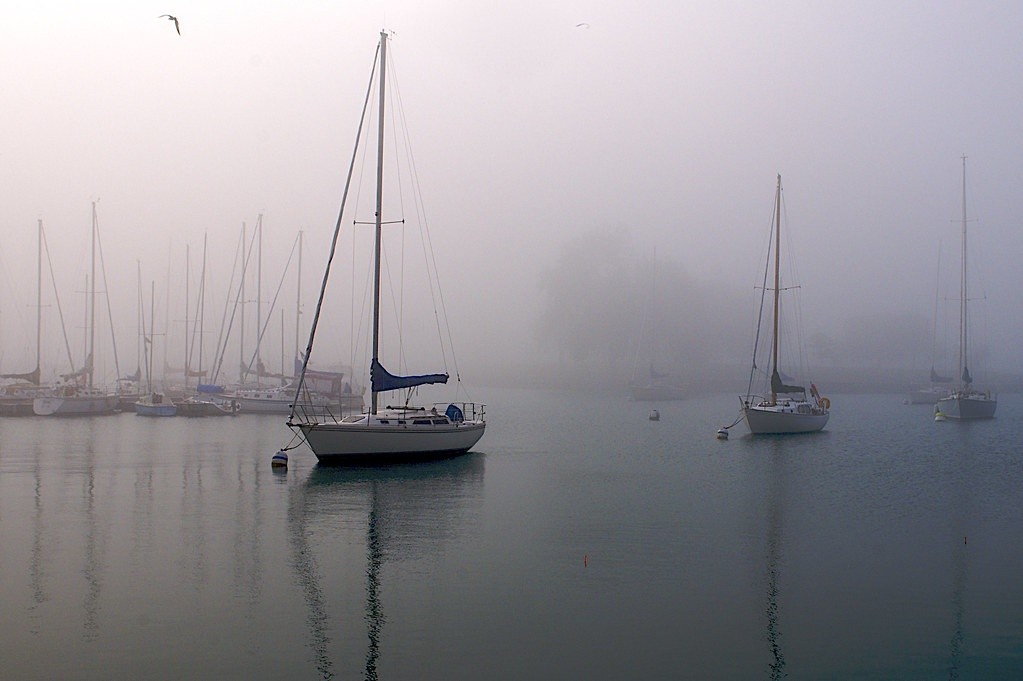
[157,14,181,37]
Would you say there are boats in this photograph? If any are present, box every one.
[906,362,953,404]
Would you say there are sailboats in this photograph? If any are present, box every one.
[936,153,1001,420]
[0,201,367,418]
[284,28,488,461]
[738,174,833,436]
